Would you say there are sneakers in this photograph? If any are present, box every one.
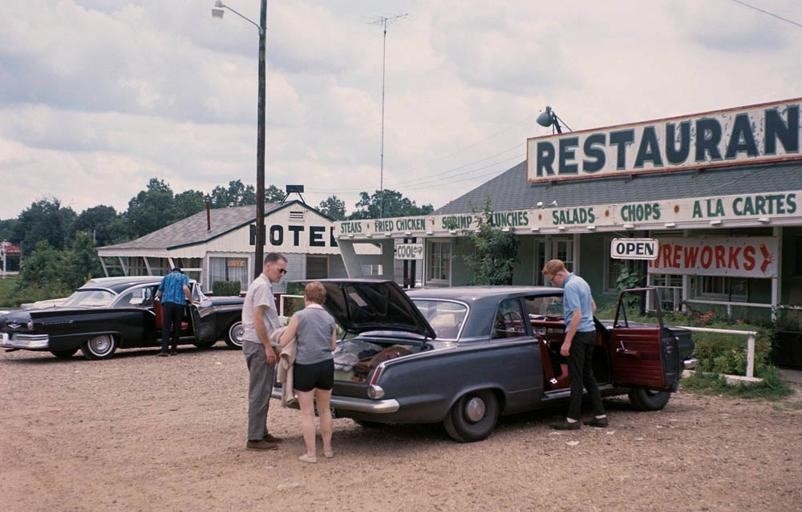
[247,434,281,450]
[298,455,318,462]
[324,451,334,457]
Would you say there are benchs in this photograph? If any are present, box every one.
[126,293,188,331]
[430,312,526,340]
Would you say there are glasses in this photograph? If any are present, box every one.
[279,268,287,274]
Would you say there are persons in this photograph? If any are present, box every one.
[241,252,288,452]
[155,268,199,356]
[541,258,609,429]
[269,281,338,464]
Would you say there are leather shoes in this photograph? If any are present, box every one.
[583,417,608,426]
[549,416,580,429]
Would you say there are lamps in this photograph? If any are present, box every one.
[536,107,573,135]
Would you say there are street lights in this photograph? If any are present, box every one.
[210,0,269,308]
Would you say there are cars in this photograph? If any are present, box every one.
[0,273,253,364]
[20,283,153,310]
[270,278,700,445]
